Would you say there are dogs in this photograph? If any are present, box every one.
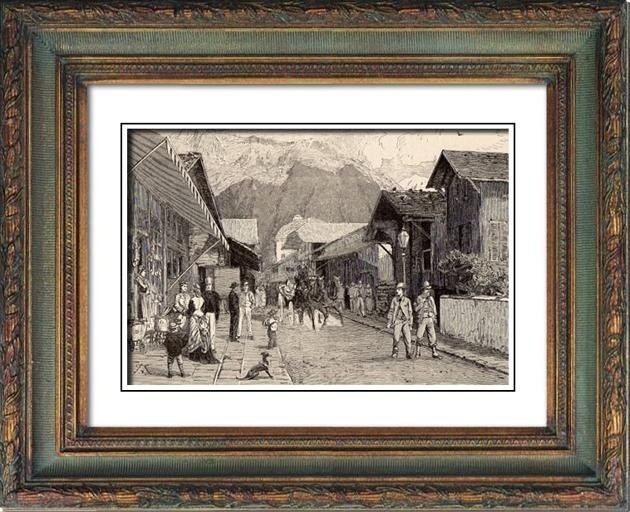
[233,352,274,380]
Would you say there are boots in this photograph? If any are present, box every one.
[168,363,174,378]
[432,345,442,359]
[392,348,399,358]
[177,363,191,377]
[406,351,414,359]
[415,345,422,358]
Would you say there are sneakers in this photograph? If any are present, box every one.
[229,335,240,342]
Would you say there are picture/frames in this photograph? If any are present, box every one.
[1,0,629,510]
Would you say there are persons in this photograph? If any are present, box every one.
[163,258,444,378]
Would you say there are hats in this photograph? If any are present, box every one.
[395,282,407,289]
[241,279,249,285]
[167,322,178,329]
[268,309,278,316]
[420,281,432,289]
[205,277,214,285]
[229,281,240,288]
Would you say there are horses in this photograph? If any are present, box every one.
[291,274,345,332]
